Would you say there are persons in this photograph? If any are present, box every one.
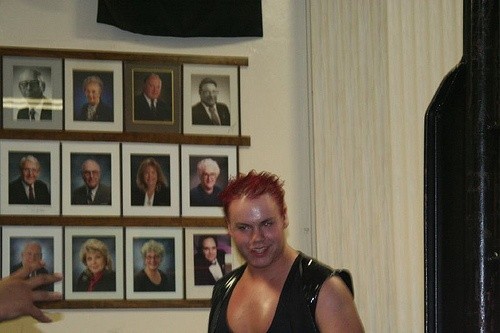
[132,158,170,206]
[17,68,53,120]
[12,241,51,290]
[192,77,231,125]
[194,235,226,285]
[208,169,366,333]
[75,239,116,292]
[0,260,64,323]
[190,158,224,207]
[79,75,114,121]
[134,72,172,121]
[71,158,111,205]
[9,154,50,204]
[135,240,172,291]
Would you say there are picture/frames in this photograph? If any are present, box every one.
[64,226,124,301]
[122,142,180,216]
[181,144,237,217]
[181,64,241,137]
[0,225,63,300]
[64,58,123,133]
[62,141,120,216]
[184,227,242,300]
[1,55,63,131]
[125,226,184,300]
[0,139,60,215]
[123,61,181,134]
